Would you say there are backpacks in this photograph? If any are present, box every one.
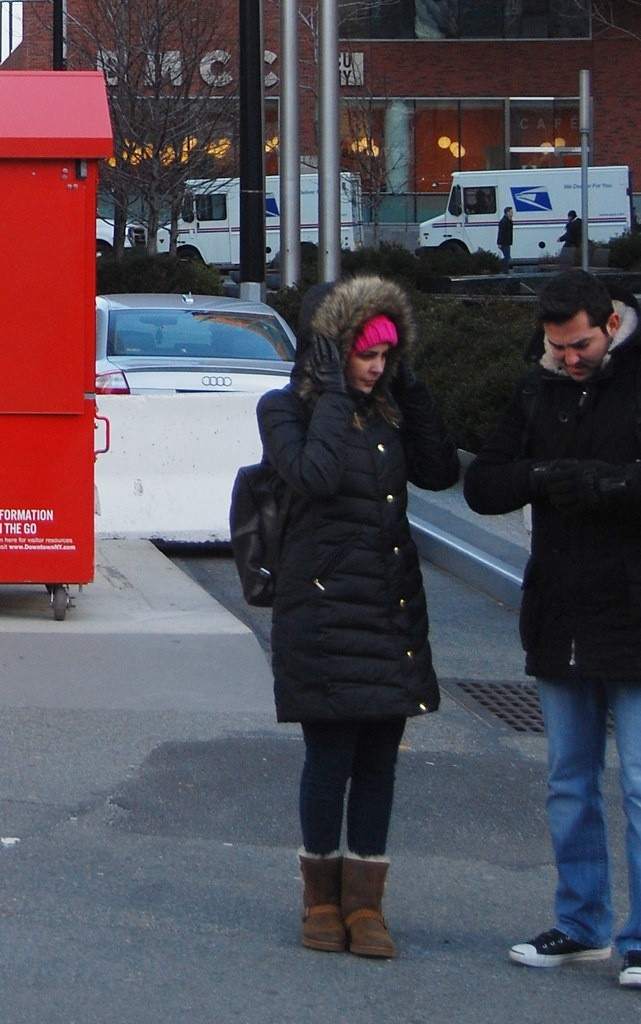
[229,389,309,607]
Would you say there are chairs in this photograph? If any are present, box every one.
[210,330,249,361]
[115,330,154,356]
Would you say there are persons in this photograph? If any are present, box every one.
[497,207,514,274]
[256,275,463,958]
[557,210,582,248]
[466,191,490,214]
[460,268,641,992]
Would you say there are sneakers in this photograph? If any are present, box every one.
[618,950,641,987]
[508,928,611,967]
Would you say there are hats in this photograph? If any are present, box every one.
[347,314,398,359]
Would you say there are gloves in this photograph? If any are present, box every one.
[394,363,416,387]
[304,333,346,393]
[529,457,585,495]
[545,470,600,514]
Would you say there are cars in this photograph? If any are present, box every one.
[94,292,302,395]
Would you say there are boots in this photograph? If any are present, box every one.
[297,845,345,951]
[340,852,394,958]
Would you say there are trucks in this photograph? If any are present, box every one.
[417,164,632,266]
[155,170,365,271]
